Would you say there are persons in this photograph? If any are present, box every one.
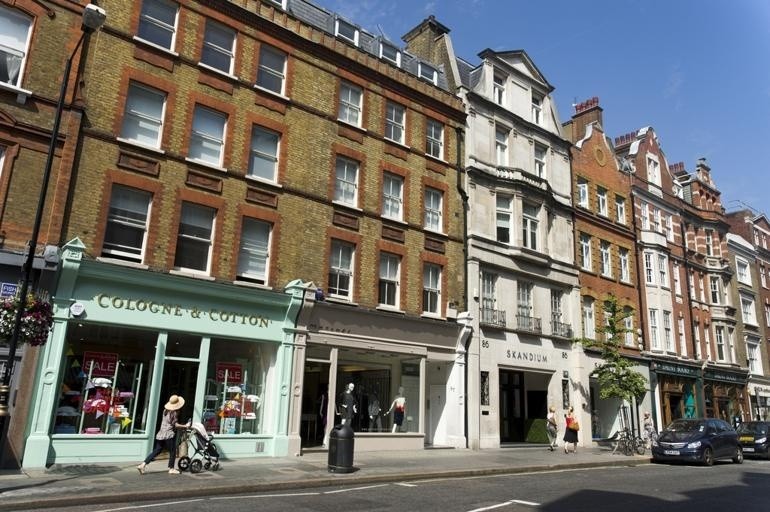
[564,406,580,453]
[135,395,191,474]
[335,383,360,433]
[319,385,340,432]
[547,406,560,450]
[383,386,408,433]
[642,411,657,441]
[367,390,384,432]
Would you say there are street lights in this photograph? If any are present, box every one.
[0,1,106,470]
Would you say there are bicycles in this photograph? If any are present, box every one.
[619,425,647,454]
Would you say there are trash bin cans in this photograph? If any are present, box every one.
[328,424,354,473]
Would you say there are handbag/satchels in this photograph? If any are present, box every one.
[569,420,579,431]
[546,418,557,426]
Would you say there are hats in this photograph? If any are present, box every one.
[164,395,185,411]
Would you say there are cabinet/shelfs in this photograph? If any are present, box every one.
[50,355,144,434]
[202,368,265,434]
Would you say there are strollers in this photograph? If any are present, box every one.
[174,421,226,472]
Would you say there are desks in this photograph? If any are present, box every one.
[301,413,318,441]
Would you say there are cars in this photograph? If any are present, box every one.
[651,417,745,465]
[734,420,769,458]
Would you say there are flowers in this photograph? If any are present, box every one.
[1,293,57,348]
